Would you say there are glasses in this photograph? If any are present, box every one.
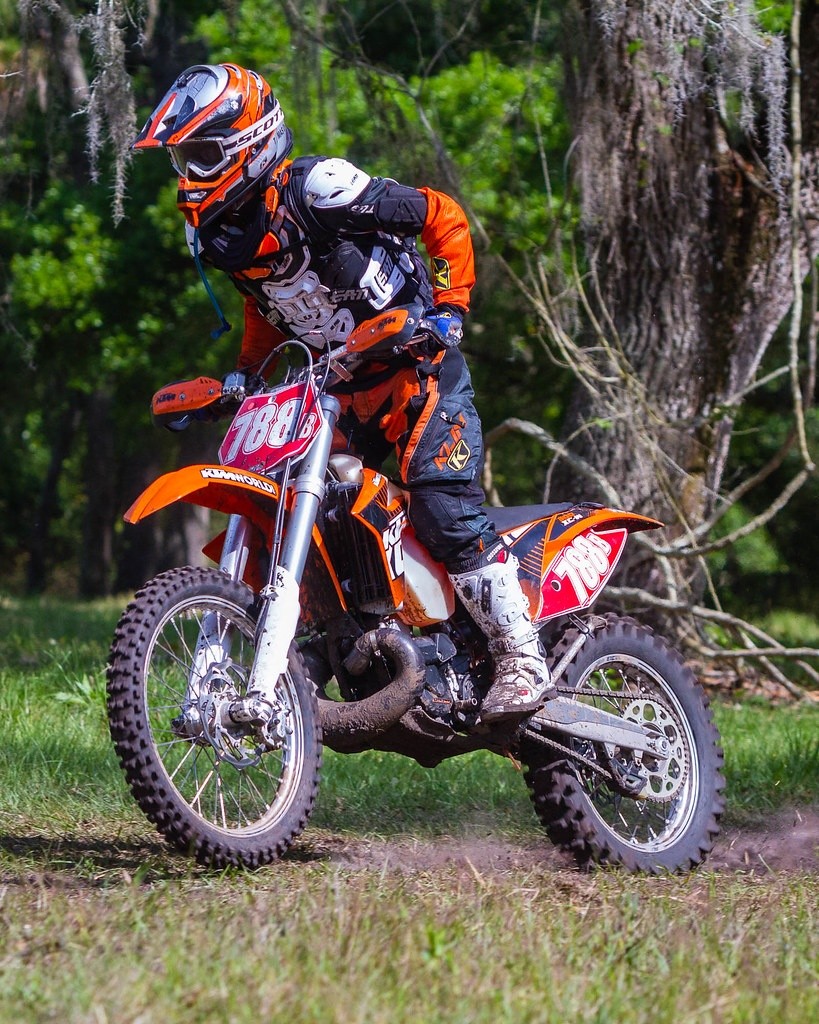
[166,136,232,179]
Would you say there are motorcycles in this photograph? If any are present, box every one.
[106,307,726,874]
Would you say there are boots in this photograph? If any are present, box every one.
[448,553,557,718]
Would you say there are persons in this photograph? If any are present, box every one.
[126,61,560,721]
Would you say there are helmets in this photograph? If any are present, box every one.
[128,64,293,229]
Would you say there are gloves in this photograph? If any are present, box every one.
[418,307,463,351]
[218,374,258,401]
